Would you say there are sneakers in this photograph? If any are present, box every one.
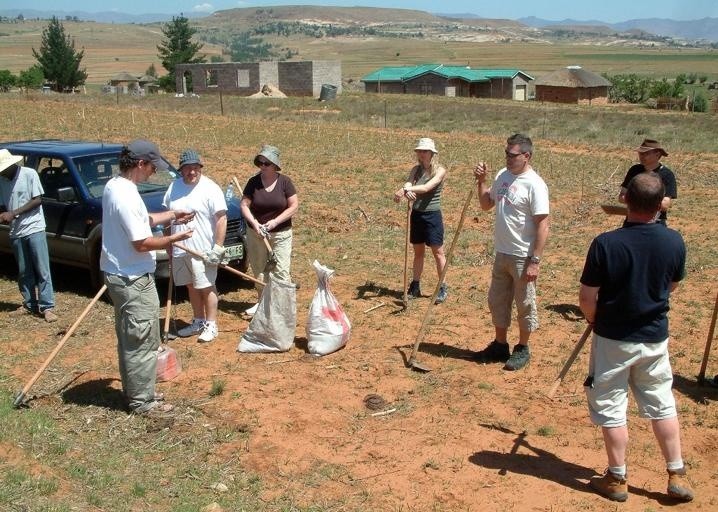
[245,302,259,316]
[45,312,58,323]
[178,322,205,336]
[155,392,164,401]
[198,323,218,342]
[590,467,628,502]
[472,339,510,363]
[401,288,421,299]
[505,344,530,370]
[9,306,29,318]
[435,287,448,305]
[144,403,174,415]
[667,469,695,501]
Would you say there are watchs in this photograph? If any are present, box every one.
[402,185,407,192]
[530,256,541,264]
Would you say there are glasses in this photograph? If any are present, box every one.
[257,161,273,167]
[505,149,526,158]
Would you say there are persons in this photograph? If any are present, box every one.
[163,149,227,342]
[1,149,58,323]
[578,172,696,500]
[240,144,297,315]
[474,134,548,370]
[618,138,677,228]
[100,138,195,414]
[394,136,448,303]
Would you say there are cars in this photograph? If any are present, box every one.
[0,139,248,304]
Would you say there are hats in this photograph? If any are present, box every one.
[253,145,282,172]
[0,149,23,172]
[176,151,204,171]
[634,138,669,156]
[121,140,169,172]
[414,138,439,154]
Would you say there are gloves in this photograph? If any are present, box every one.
[207,244,226,265]
